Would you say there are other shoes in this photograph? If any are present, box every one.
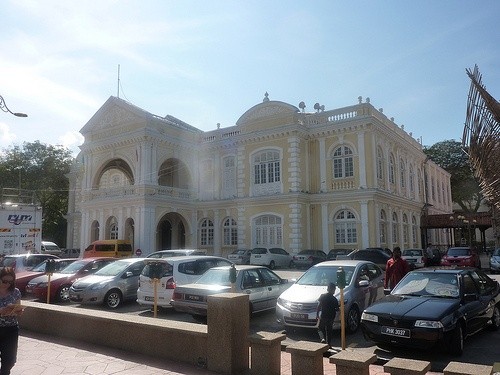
[321,338,326,343]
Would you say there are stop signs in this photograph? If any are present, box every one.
[136,249,141,256]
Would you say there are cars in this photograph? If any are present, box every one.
[0,254,82,298]
[293,250,327,269]
[400,249,426,267]
[169,264,297,321]
[440,247,481,269]
[360,265,500,355]
[323,249,353,261]
[335,249,391,271]
[275,259,390,334]
[135,249,237,308]
[25,256,120,303]
[68,258,147,309]
[489,248,500,274]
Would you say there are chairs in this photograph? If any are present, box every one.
[247,276,257,284]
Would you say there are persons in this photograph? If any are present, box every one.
[427,243,435,267]
[384,247,411,293]
[316,282,340,349]
[0,267,24,375]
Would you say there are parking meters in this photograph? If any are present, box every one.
[45,258,55,304]
[149,263,162,318]
[229,264,237,292]
[336,267,346,350]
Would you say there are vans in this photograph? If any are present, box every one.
[227,247,294,269]
[40,240,132,259]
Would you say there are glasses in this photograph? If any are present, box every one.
[1,278,14,284]
